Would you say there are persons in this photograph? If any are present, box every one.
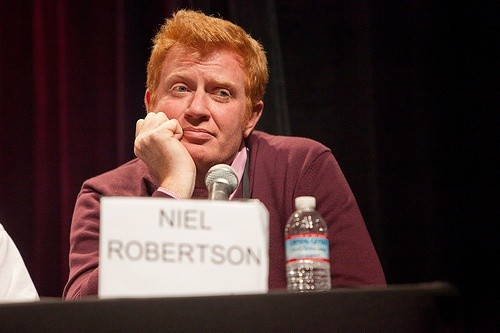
[61,10,390,301]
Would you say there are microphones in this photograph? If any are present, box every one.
[205,164,238,200]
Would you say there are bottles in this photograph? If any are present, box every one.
[285,195,333,292]
